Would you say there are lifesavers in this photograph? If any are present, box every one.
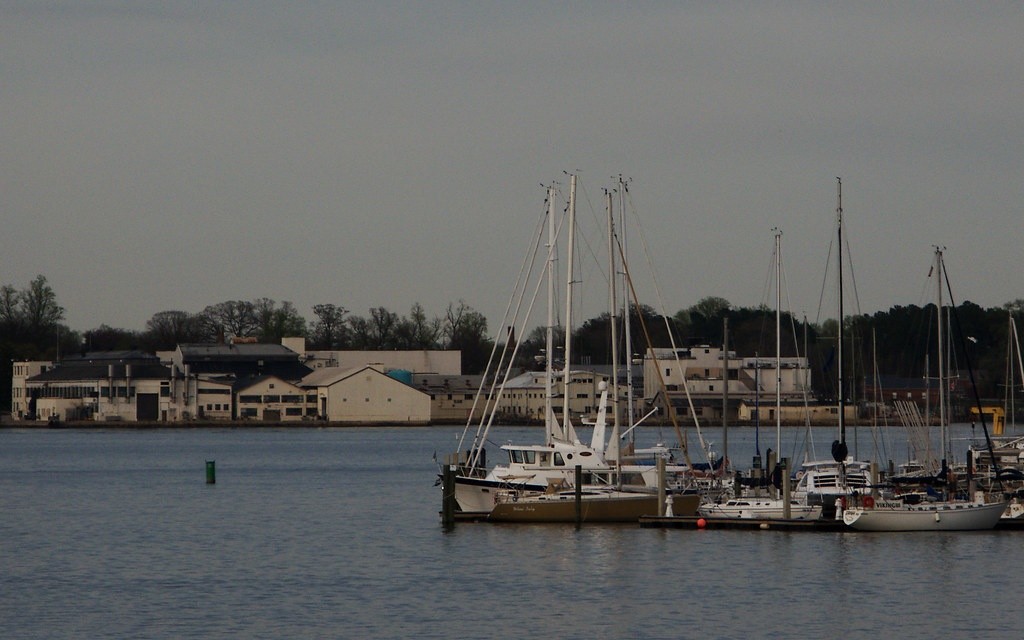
[863,496,875,508]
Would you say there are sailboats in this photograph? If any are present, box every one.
[432,168,1023,532]
